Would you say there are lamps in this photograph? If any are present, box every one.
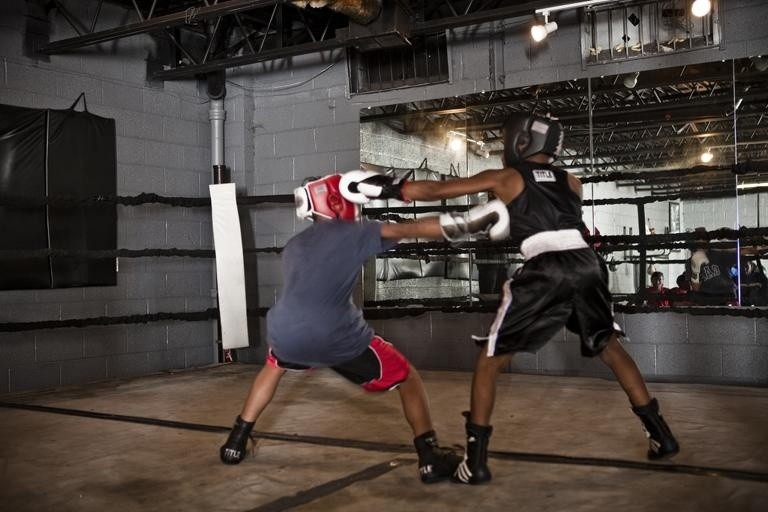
[531,10,559,43]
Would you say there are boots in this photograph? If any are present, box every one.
[629,397,680,461]
[219,414,256,465]
[413,421,493,484]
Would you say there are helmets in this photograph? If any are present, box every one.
[293,173,359,223]
[501,112,564,168]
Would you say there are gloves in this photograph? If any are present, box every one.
[338,169,413,204]
[439,200,511,244]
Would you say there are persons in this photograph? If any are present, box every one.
[219,173,511,484]
[338,111,679,484]
[644,258,768,306]
